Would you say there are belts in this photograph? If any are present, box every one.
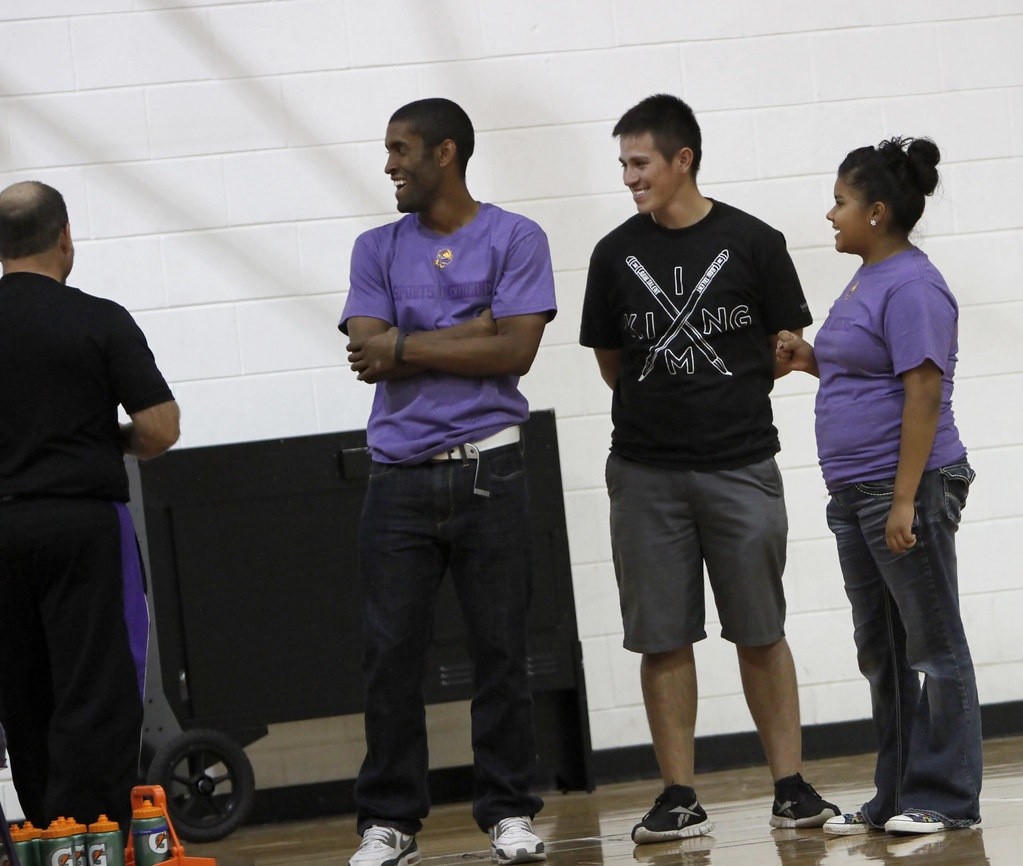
[431,424,520,496]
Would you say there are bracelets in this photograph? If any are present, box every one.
[394,331,410,367]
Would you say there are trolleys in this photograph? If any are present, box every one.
[139,407,597,846]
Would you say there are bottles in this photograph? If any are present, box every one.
[131,800,169,866]
[9,814,124,866]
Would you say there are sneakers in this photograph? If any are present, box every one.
[631,792,713,844]
[769,779,841,829]
[823,811,881,835]
[348,824,421,866]
[488,814,546,864]
[885,812,946,834]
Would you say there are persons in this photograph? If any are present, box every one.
[0,181,180,866]
[580,93,841,844]
[338,98,558,866]
[776,134,987,836]
